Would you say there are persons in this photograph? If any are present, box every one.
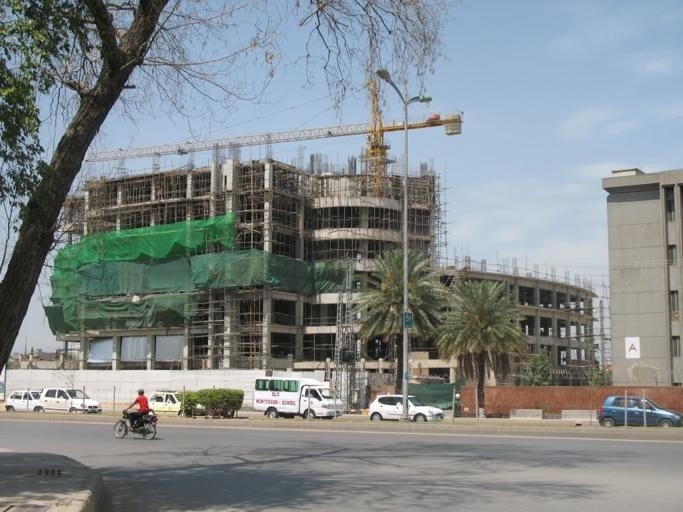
[125,389,149,427]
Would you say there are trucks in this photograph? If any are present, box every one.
[252,375,344,420]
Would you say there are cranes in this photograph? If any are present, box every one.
[85,81,464,179]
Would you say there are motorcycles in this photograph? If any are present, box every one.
[112,409,159,440]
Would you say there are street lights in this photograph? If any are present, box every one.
[375,66,433,424]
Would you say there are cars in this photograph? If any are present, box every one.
[148,390,207,418]
[595,394,683,429]
[367,394,444,423]
[4,387,103,414]
